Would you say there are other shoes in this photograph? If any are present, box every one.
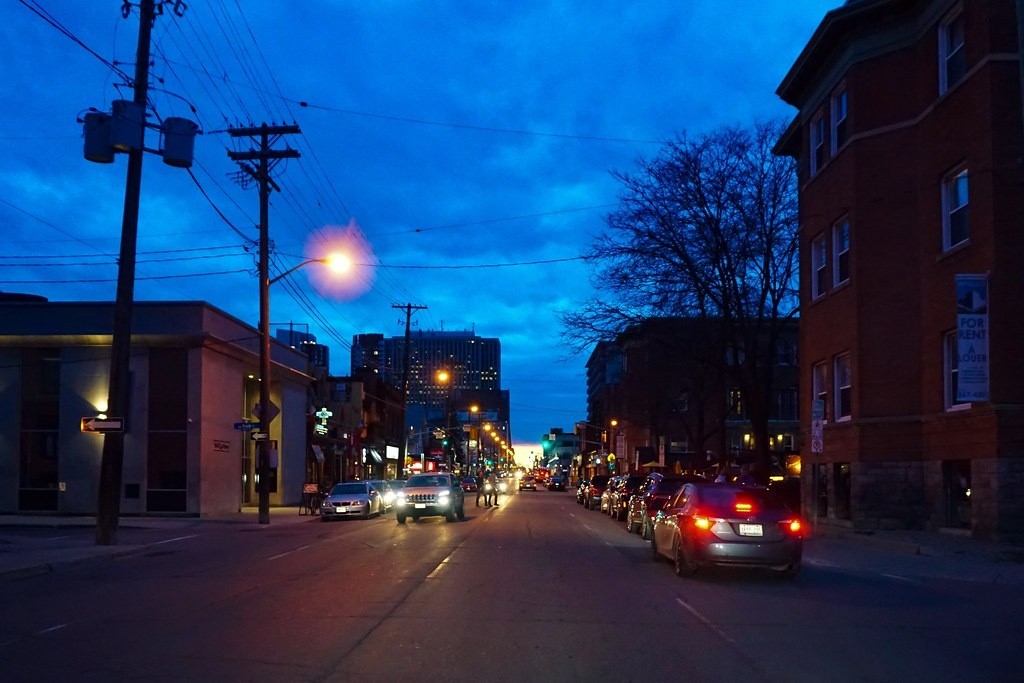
[485,504,488,506]
[476,505,480,507]
[494,504,499,506]
[488,503,492,506]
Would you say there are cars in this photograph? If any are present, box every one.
[600,476,623,515]
[543,475,567,491]
[583,475,614,511]
[395,472,464,523]
[576,480,590,505]
[320,482,383,521]
[610,473,648,522]
[651,482,804,580]
[362,480,407,514]
[519,476,537,491]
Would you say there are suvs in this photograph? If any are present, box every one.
[625,472,720,540]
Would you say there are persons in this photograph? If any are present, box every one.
[485,471,500,507]
[475,471,487,506]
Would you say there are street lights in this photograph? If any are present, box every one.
[442,406,477,472]
[396,370,447,482]
[259,255,350,525]
[466,422,514,477]
